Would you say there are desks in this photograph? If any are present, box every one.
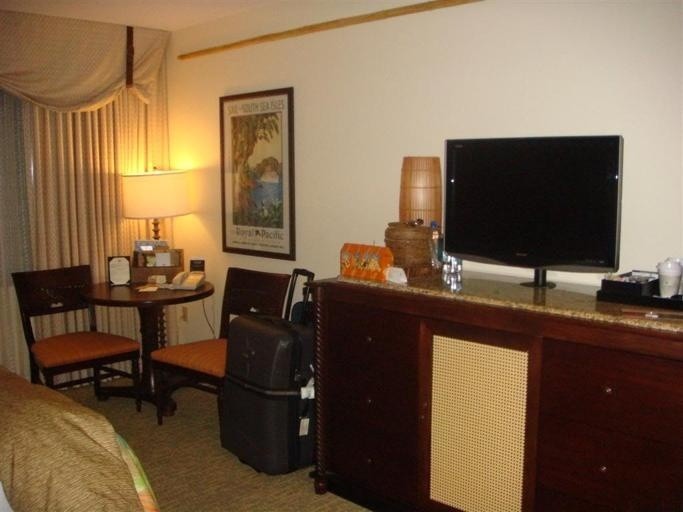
[82,282,216,416]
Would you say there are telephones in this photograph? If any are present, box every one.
[172,271,203,290]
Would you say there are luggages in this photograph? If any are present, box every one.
[218,268,317,474]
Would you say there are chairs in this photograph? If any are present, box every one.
[151,266,291,426]
[11,264,143,415]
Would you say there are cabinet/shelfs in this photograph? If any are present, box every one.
[303,288,683,512]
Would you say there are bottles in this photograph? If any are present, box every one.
[430,221,443,272]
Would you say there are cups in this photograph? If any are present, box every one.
[657,258,682,298]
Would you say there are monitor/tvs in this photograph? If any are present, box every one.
[443,134,624,290]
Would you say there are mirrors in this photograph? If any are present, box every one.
[107,256,132,288]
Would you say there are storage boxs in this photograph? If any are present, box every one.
[128,248,183,286]
[384,219,433,276]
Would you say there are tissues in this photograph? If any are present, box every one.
[339,243,406,284]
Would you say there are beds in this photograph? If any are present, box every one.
[0,364,159,512]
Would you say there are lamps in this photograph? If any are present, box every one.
[400,156,443,232]
[119,167,201,239]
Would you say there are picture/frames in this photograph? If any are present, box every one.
[218,86,296,263]
